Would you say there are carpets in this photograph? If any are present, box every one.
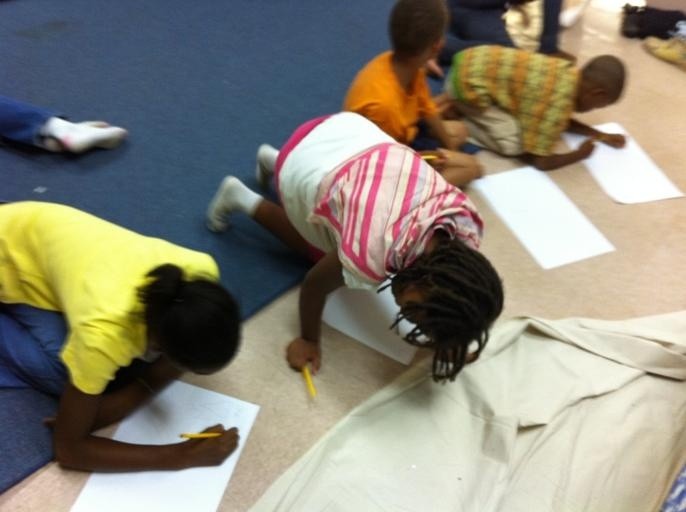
[0,1,483,491]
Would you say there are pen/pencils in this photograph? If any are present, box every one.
[180,433,222,439]
[421,155,438,160]
[302,366,316,397]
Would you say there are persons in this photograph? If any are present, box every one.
[445,44,626,170]
[0,200,241,473]
[0,95,127,153]
[208,111,503,385]
[342,0,483,188]
[425,0,576,81]
